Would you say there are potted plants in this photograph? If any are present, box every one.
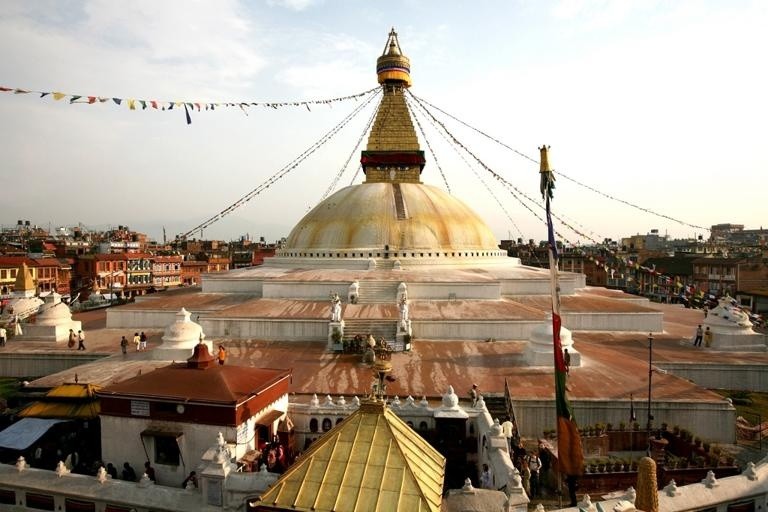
[542,421,737,477]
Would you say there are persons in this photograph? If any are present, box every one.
[260,440,286,472]
[134,333,141,351]
[182,471,198,489]
[704,327,711,346]
[397,294,409,322]
[216,346,225,365]
[121,336,129,353]
[693,325,704,346]
[330,293,342,322]
[94,460,156,486]
[78,331,86,350]
[563,349,571,374]
[0,285,14,347]
[68,329,77,348]
[141,332,146,350]
[469,385,551,499]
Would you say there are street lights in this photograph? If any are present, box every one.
[645,332,668,433]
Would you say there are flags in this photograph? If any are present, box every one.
[546,198,584,478]
